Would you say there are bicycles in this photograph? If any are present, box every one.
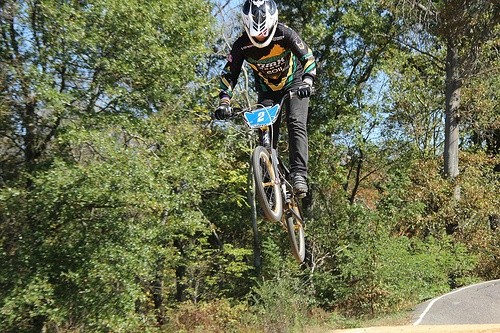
[208,84,317,261]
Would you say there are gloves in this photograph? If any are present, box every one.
[297,82,311,101]
[214,104,232,121]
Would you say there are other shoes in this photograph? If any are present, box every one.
[292,176,308,195]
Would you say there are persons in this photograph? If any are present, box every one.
[215,0,317,194]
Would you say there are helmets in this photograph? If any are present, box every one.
[240,0,278,48]
